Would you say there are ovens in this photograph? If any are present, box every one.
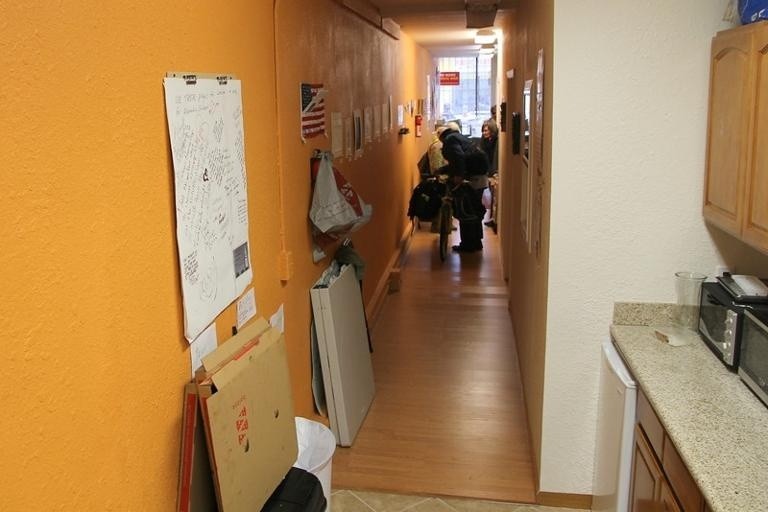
[697,278,768,373]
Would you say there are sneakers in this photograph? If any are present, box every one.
[452,240,483,253]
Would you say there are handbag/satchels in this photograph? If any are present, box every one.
[407,180,444,222]
[465,141,489,175]
[451,183,486,223]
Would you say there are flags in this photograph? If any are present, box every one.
[301,83,325,138]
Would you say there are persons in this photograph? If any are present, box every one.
[481,117,498,226]
[490,104,496,121]
[435,124,489,252]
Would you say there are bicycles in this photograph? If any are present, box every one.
[420,173,489,263]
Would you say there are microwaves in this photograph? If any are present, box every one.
[738,308,768,407]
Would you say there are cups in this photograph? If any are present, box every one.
[674,270,708,335]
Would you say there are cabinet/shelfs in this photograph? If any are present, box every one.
[629,390,718,512]
[704,21,768,257]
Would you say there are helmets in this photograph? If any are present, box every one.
[437,126,451,139]
